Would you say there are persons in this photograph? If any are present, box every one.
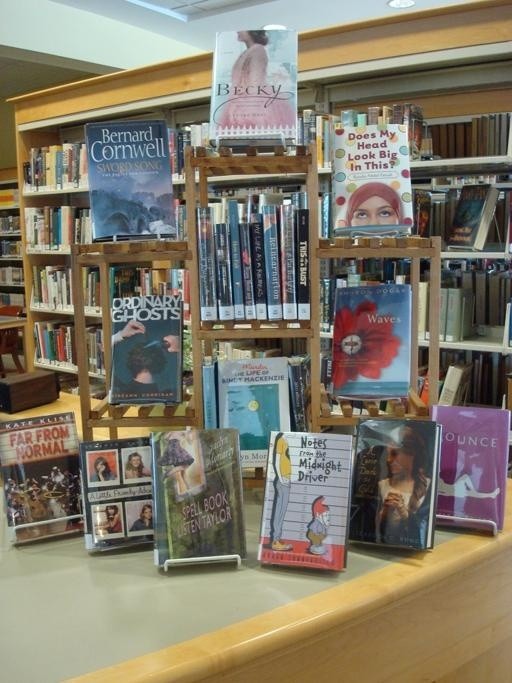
[219,29,295,134]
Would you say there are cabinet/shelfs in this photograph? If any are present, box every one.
[0,0,512,490]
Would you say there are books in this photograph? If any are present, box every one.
[206,29,301,148]
[0,99,512,574]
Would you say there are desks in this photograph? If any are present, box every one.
[0,391,512,683]
